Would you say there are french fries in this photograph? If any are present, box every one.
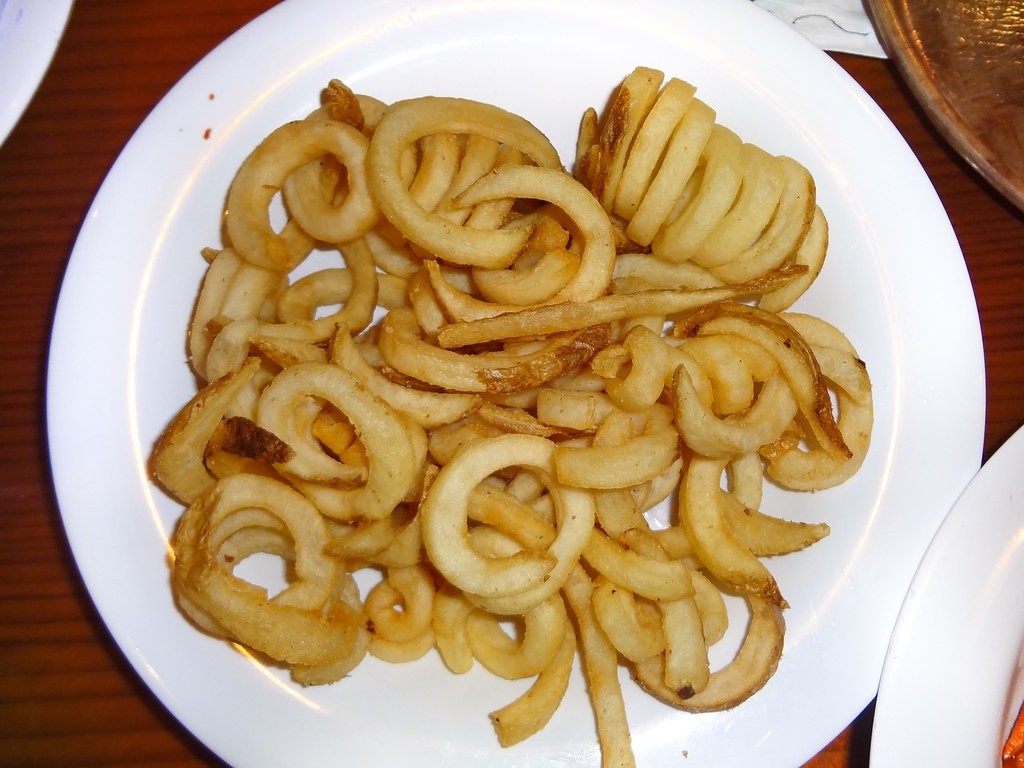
[151,68,875,767]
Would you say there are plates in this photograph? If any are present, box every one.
[867,420,1024,768]
[47,0,988,768]
[0,0,76,150]
